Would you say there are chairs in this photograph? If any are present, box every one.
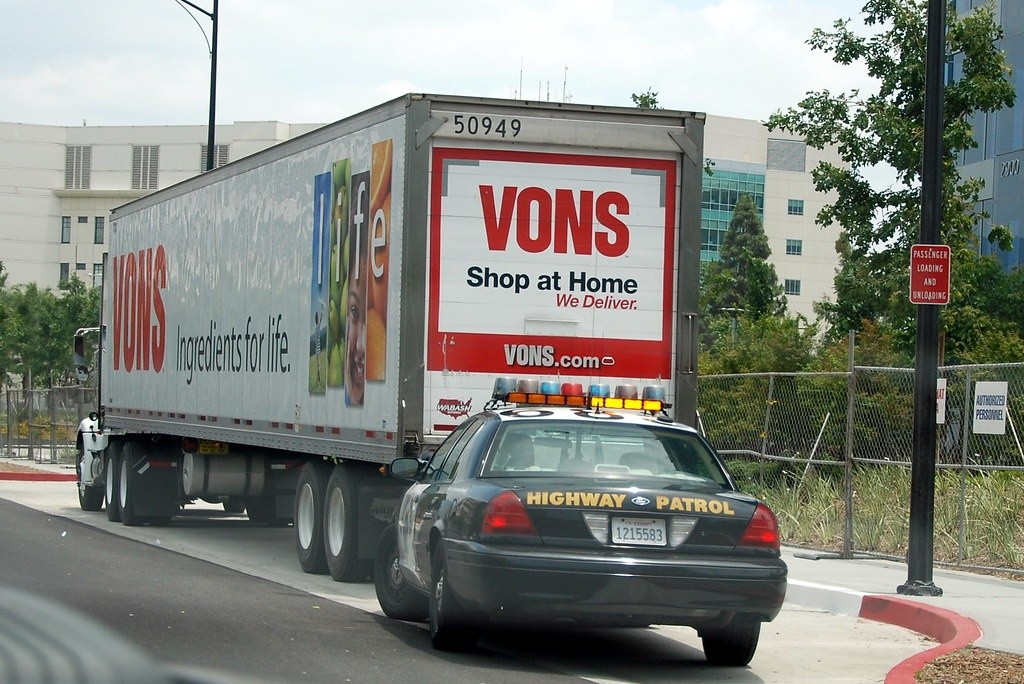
[495,433,534,470]
[619,452,662,474]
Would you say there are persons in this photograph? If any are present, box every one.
[507,433,535,468]
[314,304,324,382]
[344,170,372,407]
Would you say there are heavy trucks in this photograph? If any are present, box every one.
[71,94,708,583]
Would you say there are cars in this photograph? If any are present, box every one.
[374,378,788,671]
